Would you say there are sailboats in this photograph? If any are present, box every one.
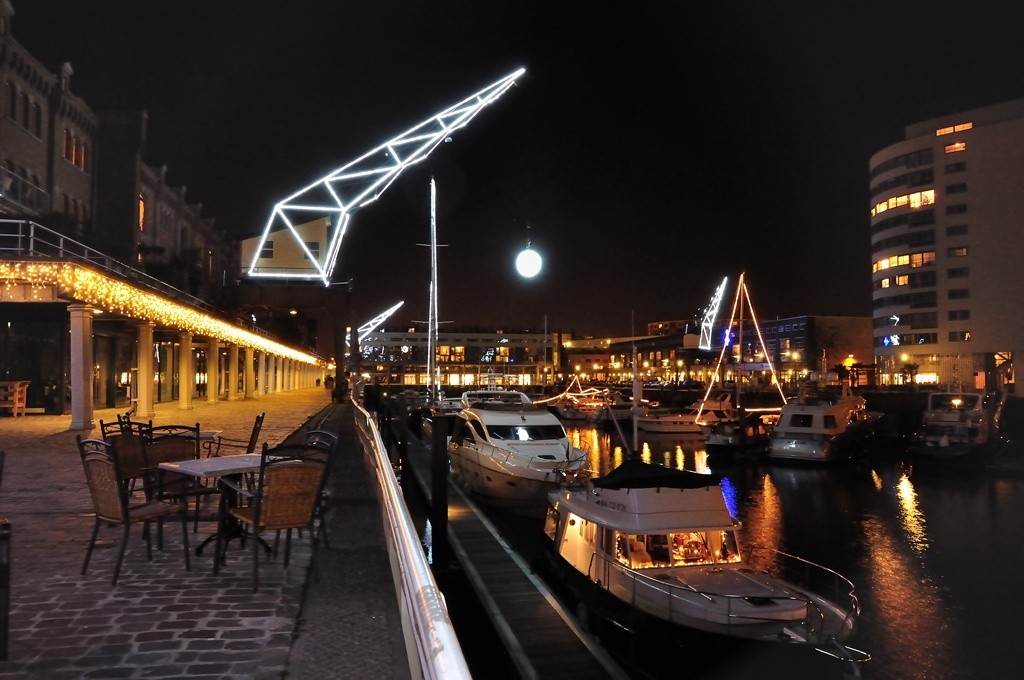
[694,272,787,446]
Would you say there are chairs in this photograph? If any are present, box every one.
[75,412,341,594]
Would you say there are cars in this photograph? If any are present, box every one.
[643,380,662,387]
[679,381,701,389]
[723,380,735,388]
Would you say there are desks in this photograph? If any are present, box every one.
[157,452,303,567]
[148,431,223,439]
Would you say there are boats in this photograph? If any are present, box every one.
[907,392,990,458]
[392,388,593,518]
[766,371,873,463]
[637,389,733,432]
[543,449,873,663]
[553,376,660,421]
